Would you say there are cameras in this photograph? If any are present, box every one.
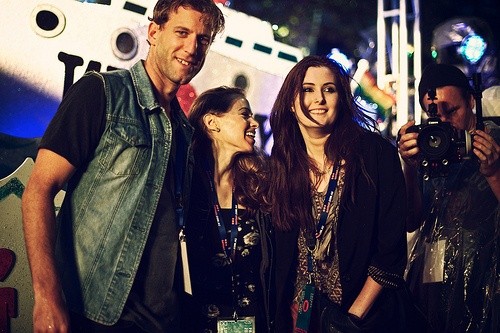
[406,87,458,160]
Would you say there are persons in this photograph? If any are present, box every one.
[188,88,273,333]
[219,55,406,333]
[21,0,224,333]
[398,64,500,333]
